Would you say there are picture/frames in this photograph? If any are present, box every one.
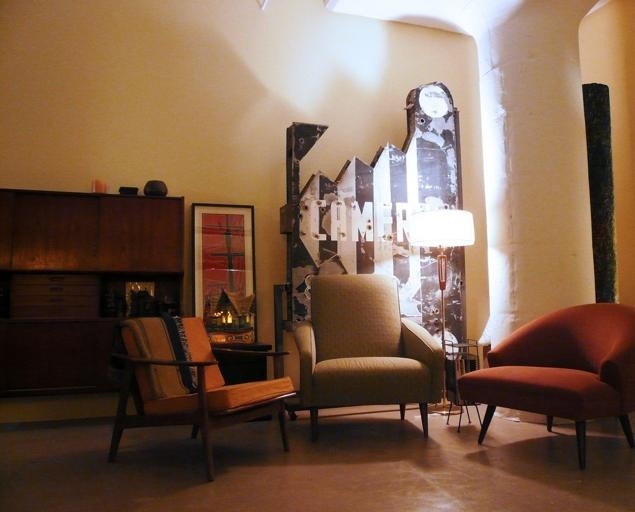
[190,201,258,346]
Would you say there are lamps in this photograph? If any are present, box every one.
[404,208,475,416]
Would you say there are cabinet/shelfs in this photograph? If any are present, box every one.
[0,188,184,400]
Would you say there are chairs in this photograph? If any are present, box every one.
[459,301,635,471]
[279,271,446,443]
[109,312,298,482]
